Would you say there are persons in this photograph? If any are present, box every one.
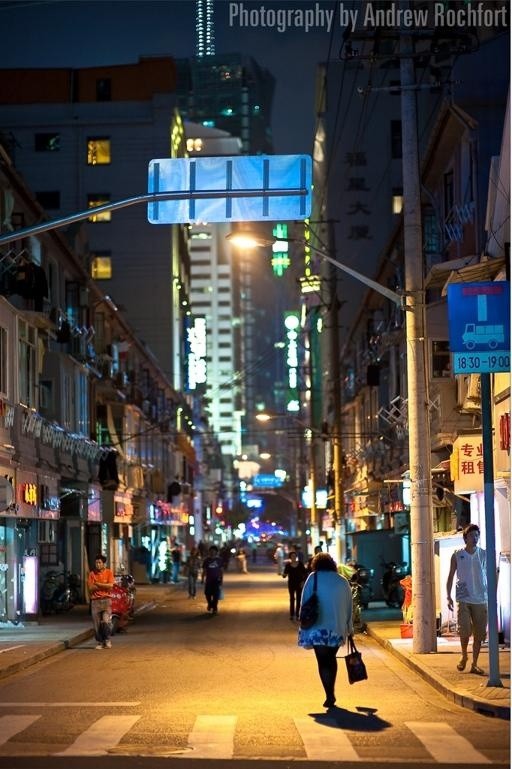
[447,525,487,674]
[88,556,116,649]
[298,553,353,707]
[172,535,323,620]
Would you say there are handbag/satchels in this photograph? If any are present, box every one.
[300,595,318,630]
[345,652,367,684]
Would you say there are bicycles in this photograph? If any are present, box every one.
[63,571,80,603]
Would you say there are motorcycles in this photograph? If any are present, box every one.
[40,567,70,617]
[347,558,374,611]
[376,551,411,610]
[106,564,134,634]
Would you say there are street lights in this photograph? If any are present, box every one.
[252,408,343,527]
[223,227,438,655]
[255,448,317,523]
